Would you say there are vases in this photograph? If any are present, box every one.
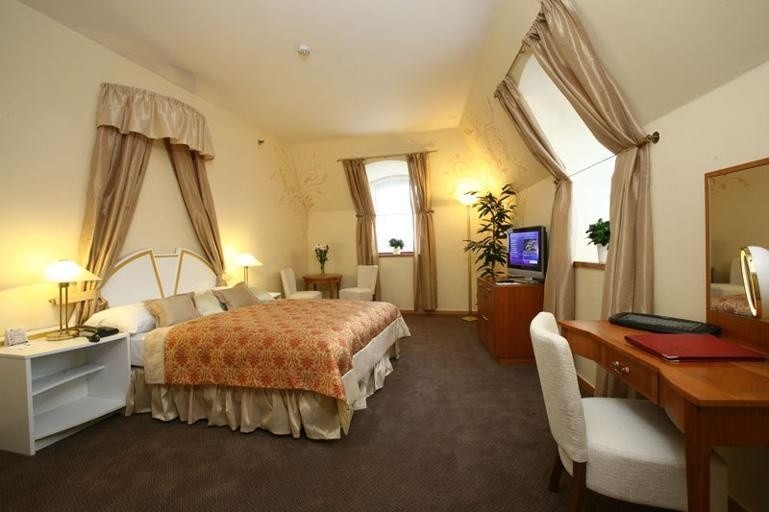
[320,264,325,276]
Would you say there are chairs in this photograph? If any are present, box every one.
[339,265,378,300]
[281,268,322,299]
[530,312,729,511]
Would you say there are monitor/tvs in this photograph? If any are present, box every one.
[507,224,549,285]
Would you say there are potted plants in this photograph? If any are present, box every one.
[389,239,404,255]
[585,218,610,264]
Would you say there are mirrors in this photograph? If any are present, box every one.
[705,158,768,349]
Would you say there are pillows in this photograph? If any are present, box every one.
[143,291,201,329]
[193,290,224,317]
[83,304,156,334]
[212,282,261,310]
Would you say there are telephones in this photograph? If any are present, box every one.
[72,326,119,342]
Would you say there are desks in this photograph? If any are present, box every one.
[303,274,342,299]
[557,320,769,512]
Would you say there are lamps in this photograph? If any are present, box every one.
[232,255,264,286]
[456,181,479,322]
[36,258,102,341]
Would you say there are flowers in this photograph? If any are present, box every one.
[313,244,329,264]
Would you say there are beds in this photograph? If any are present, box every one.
[67,248,411,440]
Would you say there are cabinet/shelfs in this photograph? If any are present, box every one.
[0,330,132,456]
[476,276,544,366]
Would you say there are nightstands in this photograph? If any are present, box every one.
[263,292,281,299]
